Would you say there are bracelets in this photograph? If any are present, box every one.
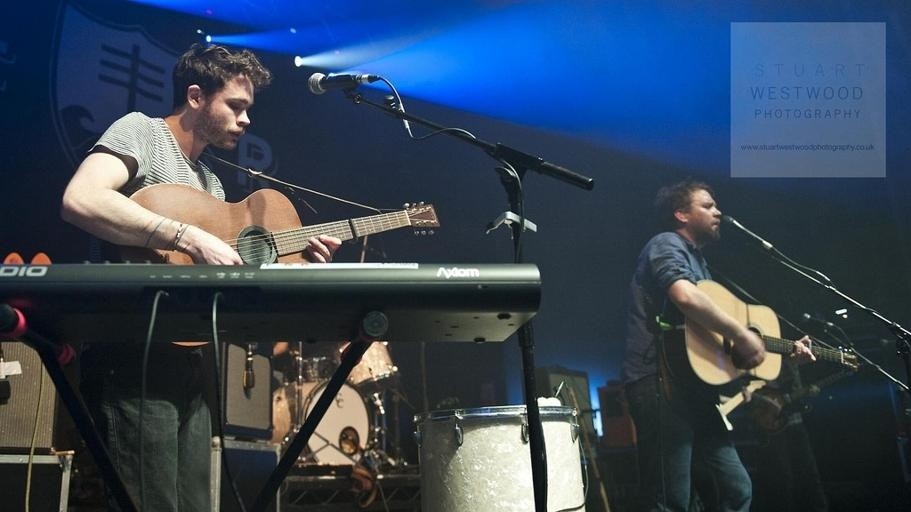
[169,220,188,250]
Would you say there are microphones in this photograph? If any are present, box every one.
[243,357,256,390]
[0,347,12,405]
[722,215,774,251]
[307,72,380,95]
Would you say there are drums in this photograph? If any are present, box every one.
[286,356,334,381]
[271,378,370,467]
[332,341,399,398]
[413,405,587,512]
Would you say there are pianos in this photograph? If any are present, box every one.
[1,261,542,344]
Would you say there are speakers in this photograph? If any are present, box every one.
[203,342,273,439]
[0,342,58,456]
[534,366,600,459]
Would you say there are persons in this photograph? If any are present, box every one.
[58,42,343,509]
[621,178,816,512]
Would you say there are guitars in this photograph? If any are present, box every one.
[127,183,442,347]
[751,368,856,434]
[673,280,857,385]
